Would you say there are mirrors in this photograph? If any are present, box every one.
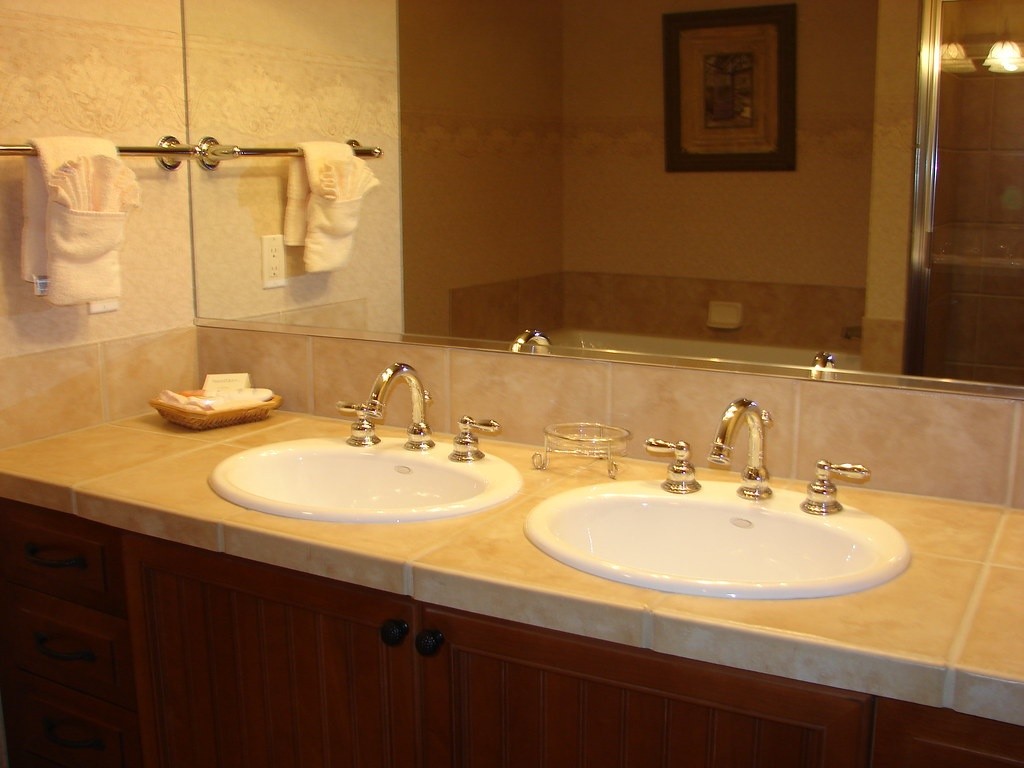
[180,0,1024,402]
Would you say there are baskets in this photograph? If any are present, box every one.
[148,388,284,431]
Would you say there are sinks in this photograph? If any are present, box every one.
[206,435,523,526]
[523,476,911,599]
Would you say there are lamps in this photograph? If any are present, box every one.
[982,19,1024,67]
[941,23,972,65]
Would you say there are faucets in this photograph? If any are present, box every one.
[362,360,436,450]
[707,398,773,499]
[511,328,553,355]
[809,351,837,368]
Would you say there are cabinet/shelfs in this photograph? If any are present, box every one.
[0,496,142,768]
[121,534,876,768]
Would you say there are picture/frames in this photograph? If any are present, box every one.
[661,4,797,173]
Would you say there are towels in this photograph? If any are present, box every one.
[20,135,141,306]
[282,140,380,274]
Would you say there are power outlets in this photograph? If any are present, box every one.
[89,300,120,315]
[262,234,286,289]
